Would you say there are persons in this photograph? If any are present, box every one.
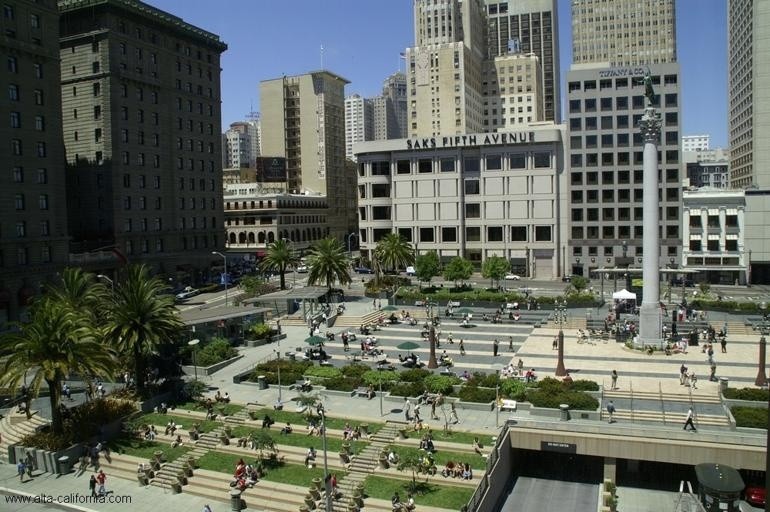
[606,401,616,423]
[86,379,484,491]
[67,386,71,400]
[97,469,107,497]
[25,450,33,478]
[306,296,558,382]
[403,492,415,512]
[609,301,729,389]
[391,491,403,512]
[62,381,67,400]
[682,404,697,430]
[88,475,98,497]
[17,457,25,481]
[203,503,212,512]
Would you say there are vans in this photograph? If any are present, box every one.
[406,267,417,276]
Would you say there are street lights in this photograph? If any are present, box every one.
[755,301,769,386]
[212,252,227,305]
[282,238,295,285]
[348,232,355,252]
[425,297,440,369]
[97,275,114,291]
[554,299,568,376]
[318,408,328,476]
[379,362,383,415]
[276,347,281,401]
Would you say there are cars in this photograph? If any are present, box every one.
[355,266,373,274]
[745,485,766,507]
[506,272,520,280]
[176,283,219,298]
[673,277,693,287]
[297,264,308,273]
[562,274,590,283]
